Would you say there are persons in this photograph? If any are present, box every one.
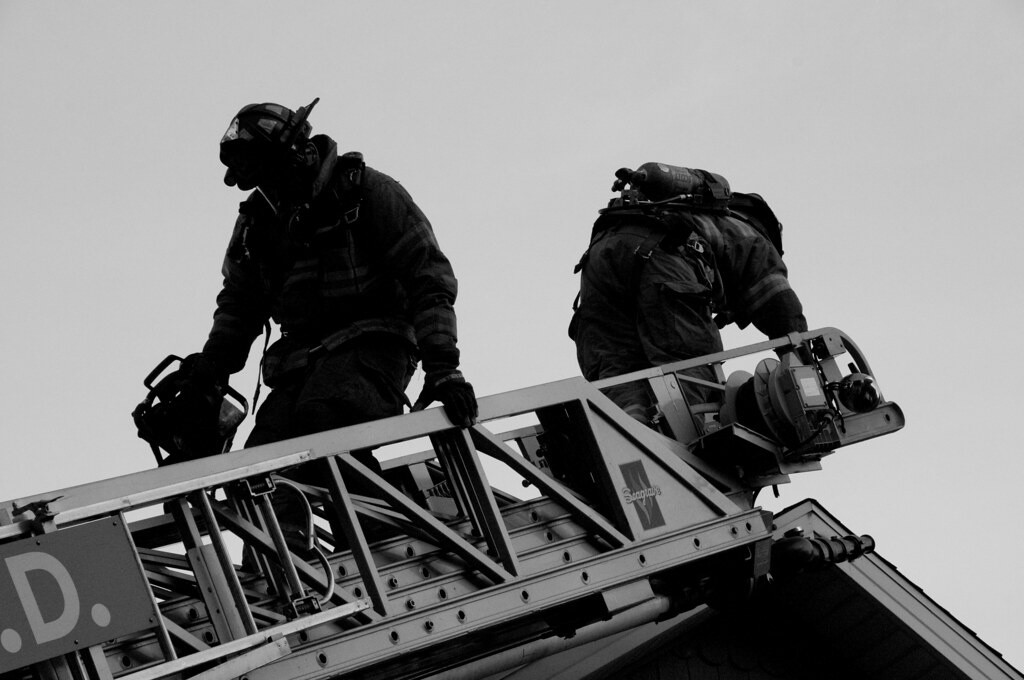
[197,101,477,570]
[569,188,809,434]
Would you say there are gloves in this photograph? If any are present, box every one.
[178,352,230,406]
[409,348,480,429]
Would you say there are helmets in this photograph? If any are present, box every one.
[219,97,320,192]
